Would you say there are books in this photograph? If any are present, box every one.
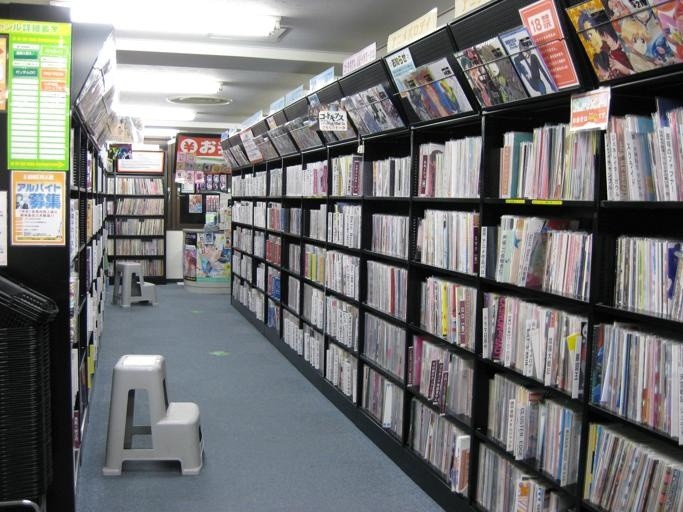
[69,122,113,492]
[230,101,681,511]
[112,173,225,286]
